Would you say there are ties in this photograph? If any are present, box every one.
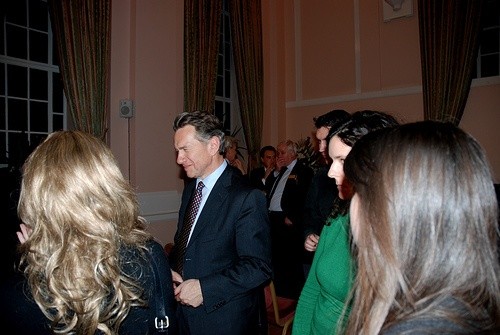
[174,181,205,287]
[266,166,288,208]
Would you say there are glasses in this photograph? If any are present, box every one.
[263,156,276,159]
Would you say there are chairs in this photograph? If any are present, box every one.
[265,281,298,335]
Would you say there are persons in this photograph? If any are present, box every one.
[224,135,315,300]
[343,121,500,335]
[167,111,273,335]
[4,129,173,335]
[304,109,355,252]
[291,111,399,335]
